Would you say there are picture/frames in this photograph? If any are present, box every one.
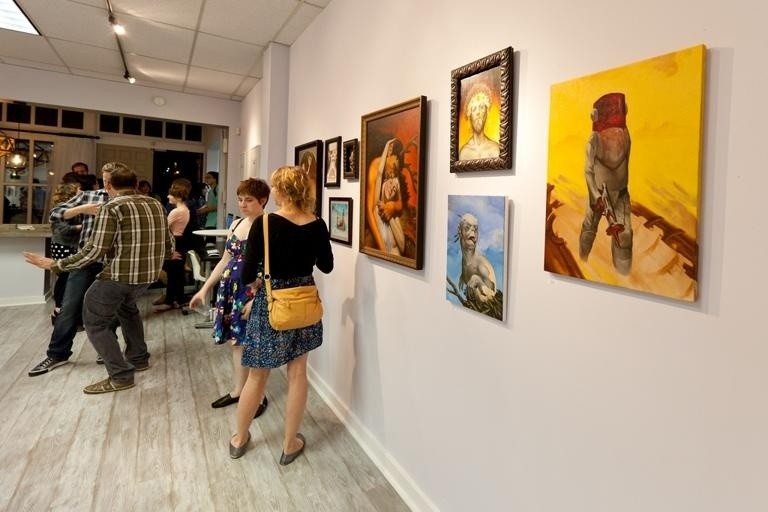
[449,46,513,172]
[293,135,359,247]
[359,95,427,271]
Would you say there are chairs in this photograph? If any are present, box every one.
[187,249,215,328]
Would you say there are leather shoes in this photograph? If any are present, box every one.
[230,430,251,459]
[254,395,268,419]
[83,378,135,395]
[279,432,305,465]
[211,394,239,408]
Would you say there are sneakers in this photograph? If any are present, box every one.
[96,353,104,363]
[29,356,69,376]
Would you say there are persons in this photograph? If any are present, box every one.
[299,153,316,180]
[27,160,139,380]
[457,81,500,161]
[19,164,174,397]
[188,175,276,419]
[4,158,220,333]
[325,142,336,183]
[373,176,406,257]
[367,135,416,258]
[227,165,336,465]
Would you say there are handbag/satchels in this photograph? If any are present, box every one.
[268,285,324,331]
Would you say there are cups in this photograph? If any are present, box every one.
[189,299,210,317]
